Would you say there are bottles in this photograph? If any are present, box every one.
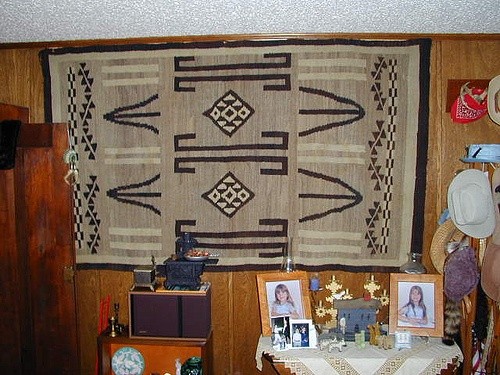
[280,237,297,273]
[399,252,425,274]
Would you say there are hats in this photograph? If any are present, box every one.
[487,75,500,125]
[429,219,487,276]
[481,240,500,301]
[447,169,495,239]
[444,246,479,301]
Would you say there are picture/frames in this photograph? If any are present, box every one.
[389,272,445,337]
[255,270,312,336]
[292,319,312,349]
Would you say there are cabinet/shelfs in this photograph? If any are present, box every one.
[128,283,213,342]
[0,103,82,375]
[97,327,213,375]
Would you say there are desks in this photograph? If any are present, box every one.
[259,332,463,375]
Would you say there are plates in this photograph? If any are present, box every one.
[184,254,208,261]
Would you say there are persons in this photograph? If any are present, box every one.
[273,320,289,349]
[293,327,309,347]
[398,286,427,324]
[270,284,299,318]
